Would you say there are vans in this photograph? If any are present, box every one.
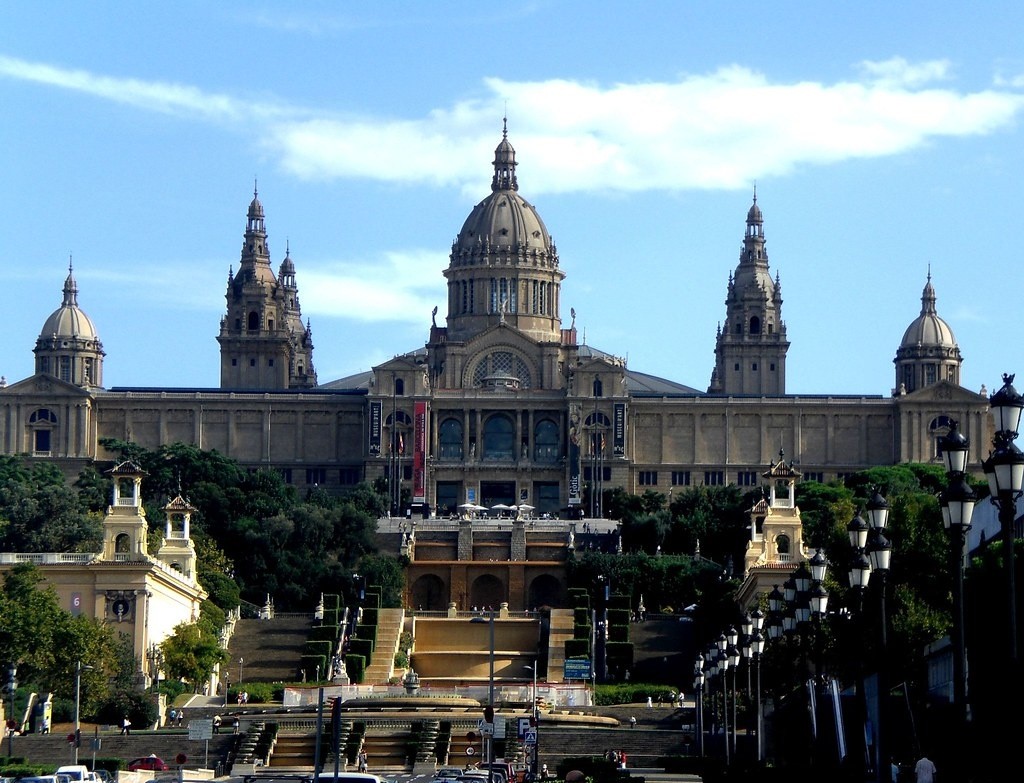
[479,762,517,783]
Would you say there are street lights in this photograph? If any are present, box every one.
[470,616,493,783]
[765,584,787,783]
[522,665,535,765]
[73,665,93,764]
[725,624,739,783]
[694,632,734,783]
[790,548,829,783]
[982,372,1024,783]
[847,483,899,783]
[742,602,763,783]
[936,420,975,783]
[7,661,18,757]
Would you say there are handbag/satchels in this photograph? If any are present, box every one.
[365,759,368,763]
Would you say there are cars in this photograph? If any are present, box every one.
[312,772,387,783]
[0,772,73,783]
[55,765,88,783]
[87,769,112,783]
[433,761,509,783]
[128,757,167,771]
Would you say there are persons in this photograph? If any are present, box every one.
[418,604,423,611]
[468,511,537,519]
[235,690,248,707]
[42,716,49,734]
[646,690,685,708]
[891,752,936,783]
[465,764,472,771]
[227,680,231,690]
[628,715,636,729]
[426,683,432,693]
[169,708,176,728]
[470,604,493,611]
[25,720,30,734]
[233,713,239,734]
[358,749,368,773]
[120,715,131,736]
[177,709,184,727]
[603,749,627,769]
[213,714,222,734]
[625,670,631,683]
[216,682,223,696]
[533,607,537,612]
[578,508,585,520]
[539,763,548,783]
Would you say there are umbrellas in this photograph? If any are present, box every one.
[458,503,535,510]
